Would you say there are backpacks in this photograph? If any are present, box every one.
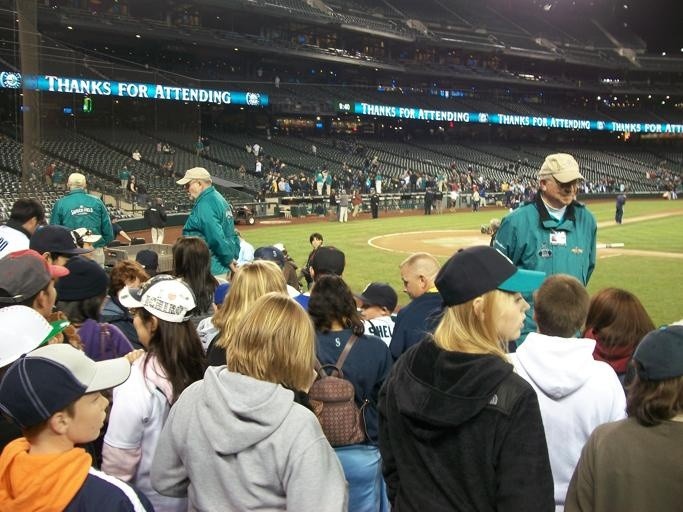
[306,362,368,449]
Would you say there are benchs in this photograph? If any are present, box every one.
[104,243,184,274]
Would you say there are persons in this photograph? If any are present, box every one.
[235,226,253,265]
[491,153,598,354]
[175,167,240,283]
[291,246,346,313]
[307,276,396,512]
[171,235,219,332]
[390,252,445,360]
[253,245,285,271]
[48,173,115,269]
[303,233,324,269]
[565,324,682,511]
[111,217,131,243]
[206,259,289,366]
[150,292,350,512]
[0,196,46,260]
[29,225,96,267]
[274,242,302,292]
[101,273,210,512]
[136,249,159,277]
[0,0,683,250]
[0,251,66,323]
[0,343,155,512]
[353,283,399,347]
[194,283,234,355]
[584,288,655,387]
[107,258,151,317]
[0,303,70,378]
[503,273,629,511]
[53,256,135,362]
[377,245,557,512]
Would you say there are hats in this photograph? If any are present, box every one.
[433,243,547,307]
[1,248,71,304]
[135,249,160,269]
[309,245,347,276]
[2,341,132,428]
[252,245,285,267]
[67,172,88,187]
[349,279,398,311]
[29,223,94,255]
[115,272,199,323]
[0,305,71,368]
[109,214,119,220]
[52,254,110,301]
[175,166,212,187]
[630,320,682,381]
[212,282,235,305]
[274,243,286,252]
[537,152,587,186]
